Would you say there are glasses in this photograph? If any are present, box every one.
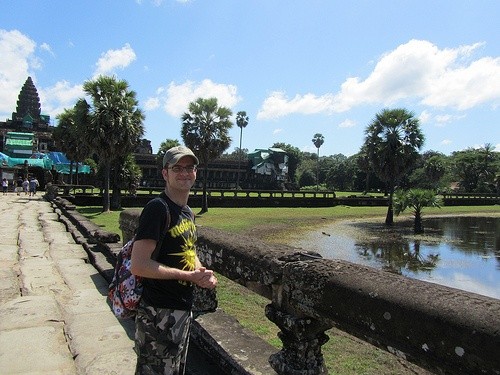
[165,166,197,173]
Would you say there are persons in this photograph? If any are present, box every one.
[3,176,39,197]
[130,146,218,375]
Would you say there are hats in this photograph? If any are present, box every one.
[163,146,199,169]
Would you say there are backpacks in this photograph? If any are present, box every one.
[108,197,194,320]
